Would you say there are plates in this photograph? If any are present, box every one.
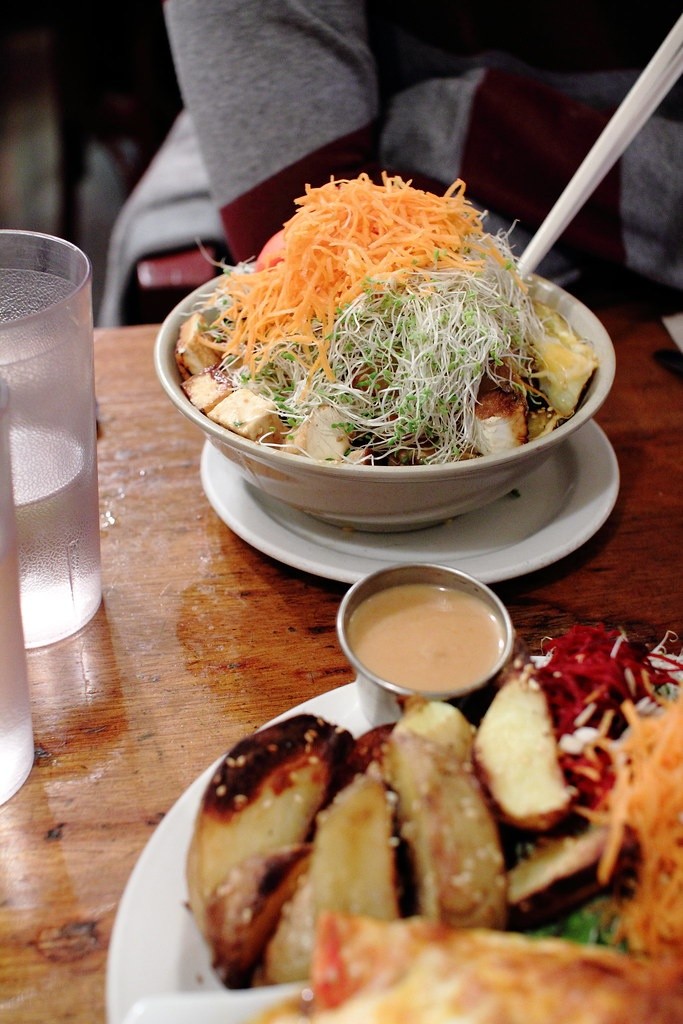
[201,419,620,586]
[104,655,683,1024]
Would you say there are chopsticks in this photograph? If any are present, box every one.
[518,12,683,279]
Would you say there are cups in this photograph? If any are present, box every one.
[0,379,35,807]
[0,229,103,656]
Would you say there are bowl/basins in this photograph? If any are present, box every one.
[336,563,514,734]
[154,261,617,534]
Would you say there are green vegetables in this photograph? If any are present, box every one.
[235,220,557,467]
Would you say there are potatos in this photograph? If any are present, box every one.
[184,668,574,993]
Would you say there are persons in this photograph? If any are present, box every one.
[164,0,682,298]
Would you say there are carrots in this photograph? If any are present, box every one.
[189,171,529,386]
[562,664,683,965]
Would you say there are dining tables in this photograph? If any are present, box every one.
[0,316,683,1024]
